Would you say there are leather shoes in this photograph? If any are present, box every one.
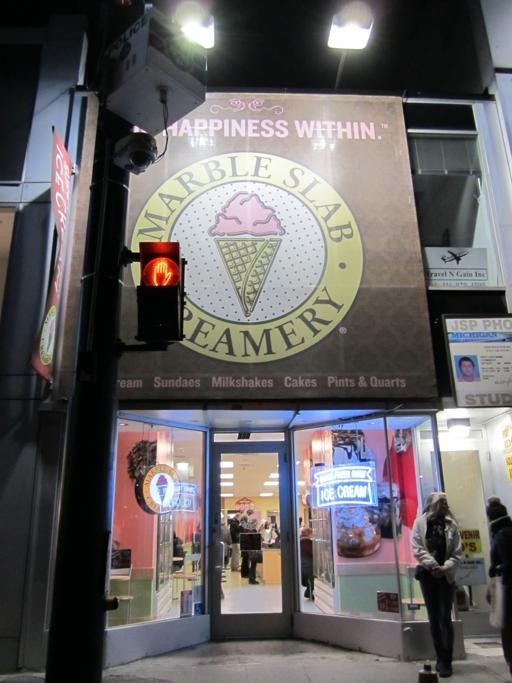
[304,592,314,600]
[434,655,453,678]
[249,579,260,584]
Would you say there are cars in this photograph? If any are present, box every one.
[331,477,404,559]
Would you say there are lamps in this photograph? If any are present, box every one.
[171,2,215,86]
[328,1,375,89]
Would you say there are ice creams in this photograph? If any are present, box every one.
[156,474,168,504]
[210,191,286,320]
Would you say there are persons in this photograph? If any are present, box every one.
[172,530,185,571]
[300,527,314,601]
[410,491,462,678]
[230,512,274,585]
[486,497,512,674]
[453,355,482,383]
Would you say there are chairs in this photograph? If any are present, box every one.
[301,537,312,602]
[401,567,426,621]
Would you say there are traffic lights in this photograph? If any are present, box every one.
[135,239,189,344]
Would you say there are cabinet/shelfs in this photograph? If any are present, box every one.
[256,548,281,584]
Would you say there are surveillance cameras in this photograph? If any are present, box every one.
[127,148,156,170]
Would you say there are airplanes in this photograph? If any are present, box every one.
[441,248,473,266]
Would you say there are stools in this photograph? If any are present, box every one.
[171,551,201,600]
[116,595,134,624]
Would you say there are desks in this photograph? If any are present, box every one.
[106,564,132,627]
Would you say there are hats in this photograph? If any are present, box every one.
[486,496,507,519]
[427,491,448,504]
[299,528,313,539]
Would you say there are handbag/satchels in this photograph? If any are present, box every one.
[414,564,428,581]
[488,575,505,629]
